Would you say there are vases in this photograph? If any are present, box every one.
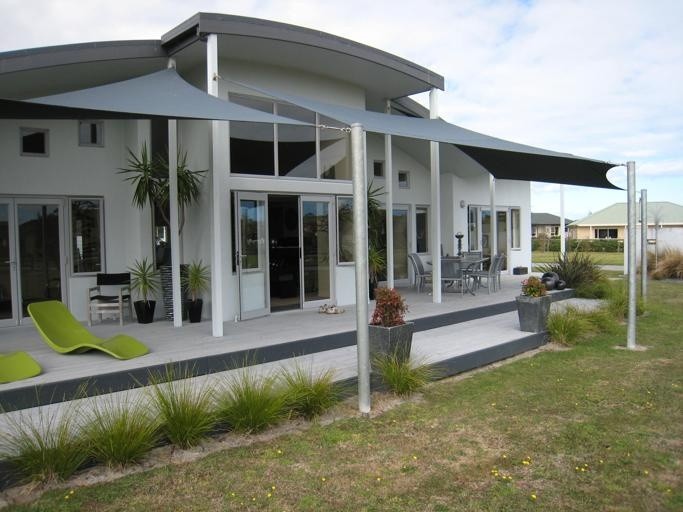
[367,322,414,373]
[516,295,551,332]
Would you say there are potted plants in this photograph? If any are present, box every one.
[114,139,210,321]
[367,176,389,300]
[122,256,163,323]
[180,259,212,322]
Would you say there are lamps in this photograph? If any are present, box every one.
[455,232,464,256]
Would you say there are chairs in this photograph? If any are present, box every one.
[408,252,503,296]
[0,351,42,383]
[27,300,149,359]
[86,272,133,327]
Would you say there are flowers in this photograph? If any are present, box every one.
[520,275,547,297]
[371,285,411,327]
